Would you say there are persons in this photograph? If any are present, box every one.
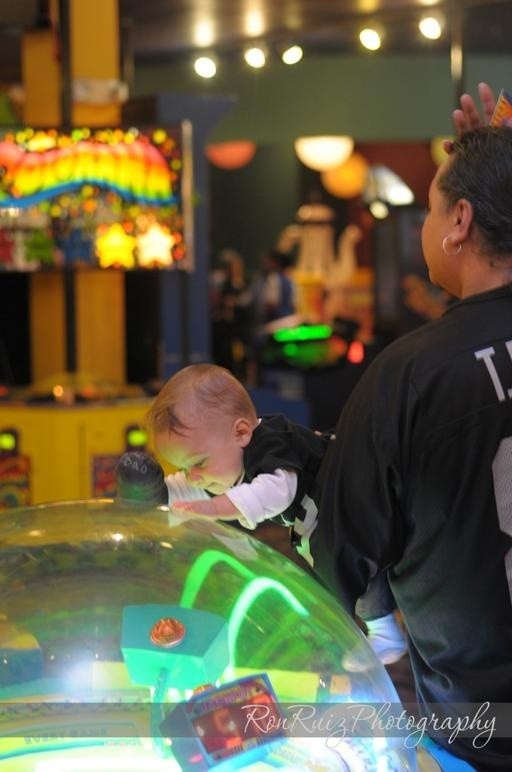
[148,362,409,673]
[314,82,512,772]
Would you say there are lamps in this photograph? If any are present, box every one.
[193,8,447,80]
